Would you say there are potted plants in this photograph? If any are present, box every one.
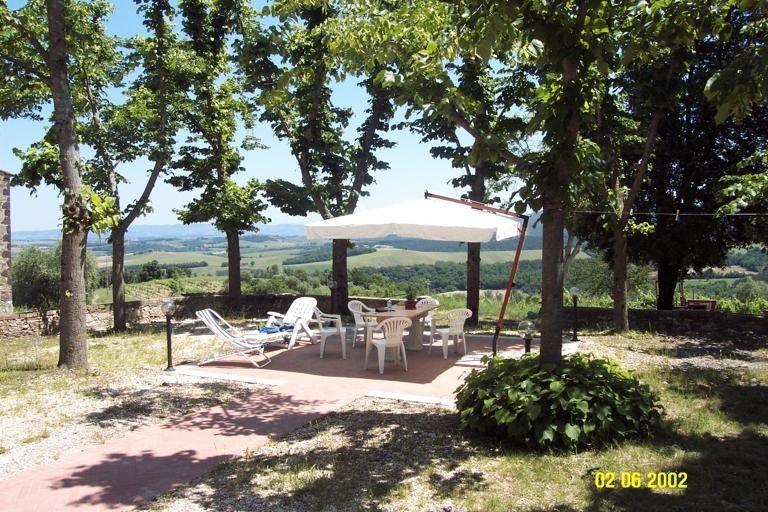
[403,285,418,309]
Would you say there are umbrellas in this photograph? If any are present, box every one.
[304,191,530,357]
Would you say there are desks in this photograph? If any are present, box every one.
[360,302,442,352]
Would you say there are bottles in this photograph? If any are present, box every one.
[387,298,392,309]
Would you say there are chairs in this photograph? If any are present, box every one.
[194,307,272,369]
[363,317,411,374]
[241,296,317,351]
[346,299,375,349]
[427,308,472,360]
[312,305,346,361]
[414,297,439,311]
